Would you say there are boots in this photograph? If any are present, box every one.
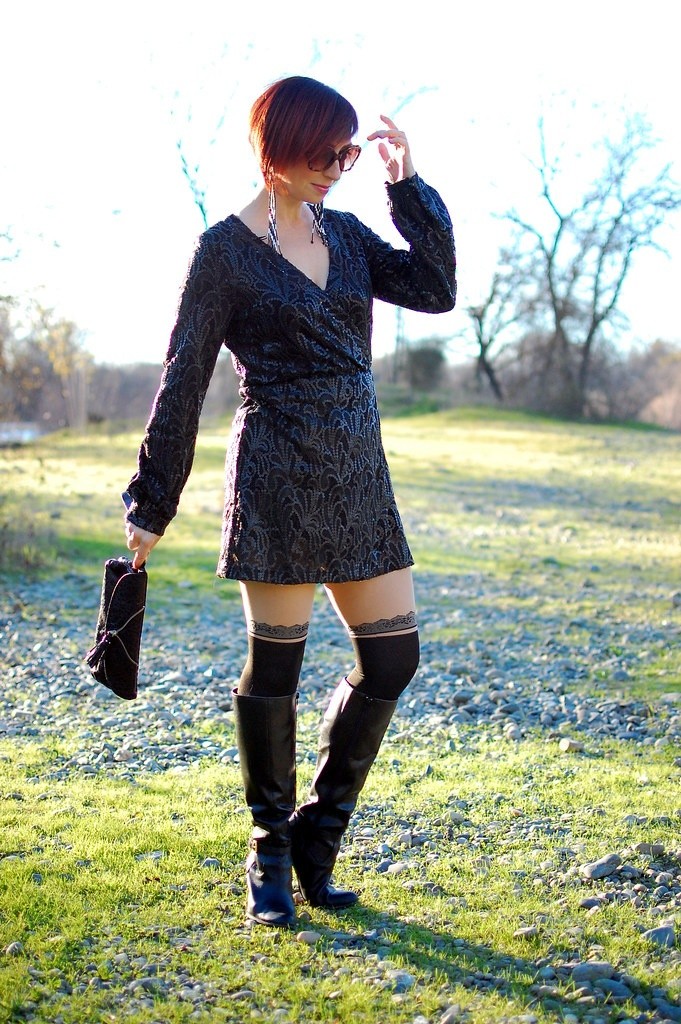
[232,686,297,929]
[289,676,399,911]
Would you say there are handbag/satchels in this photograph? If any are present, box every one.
[85,555,148,700]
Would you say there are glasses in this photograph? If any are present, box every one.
[308,144,362,173]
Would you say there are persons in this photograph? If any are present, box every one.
[124,75,457,927]
